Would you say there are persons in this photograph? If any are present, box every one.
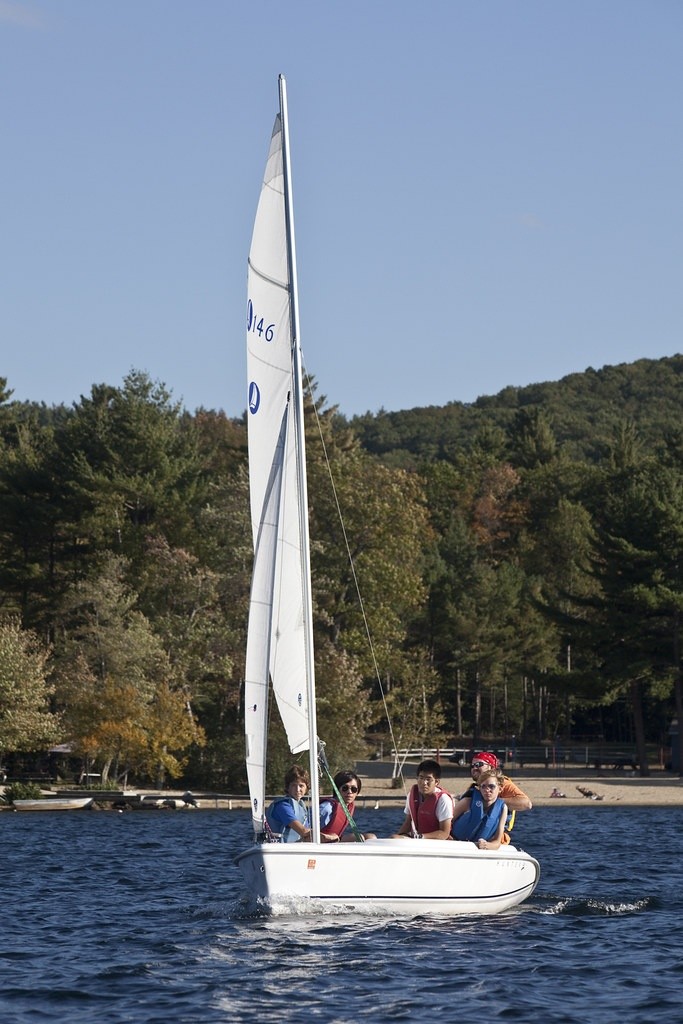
[469,752,533,811]
[266,764,339,843]
[307,770,377,842]
[550,785,602,799]
[452,769,508,851]
[389,760,454,840]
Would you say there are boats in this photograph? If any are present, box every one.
[12,797,94,811]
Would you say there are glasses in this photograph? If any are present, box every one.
[342,785,358,793]
[418,777,437,782]
[471,762,488,768]
[482,784,495,789]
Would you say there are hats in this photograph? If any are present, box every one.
[473,752,498,768]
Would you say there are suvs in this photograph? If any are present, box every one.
[447,744,512,767]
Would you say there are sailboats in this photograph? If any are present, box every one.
[223,73,542,923]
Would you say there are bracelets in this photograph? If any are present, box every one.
[419,833,422,839]
